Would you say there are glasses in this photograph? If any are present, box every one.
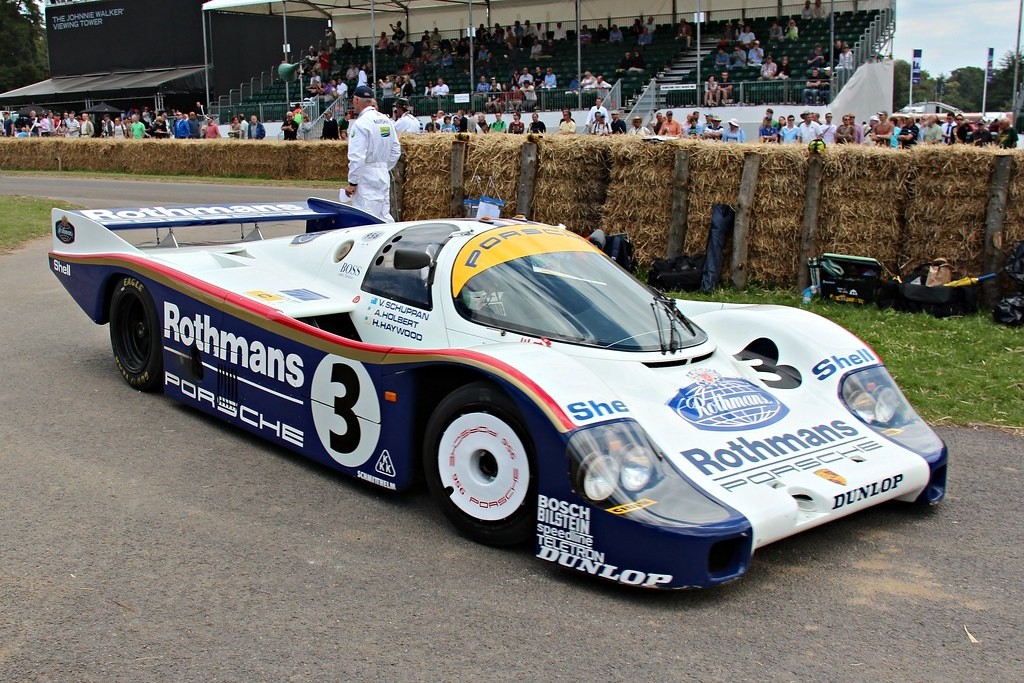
[789,114,856,122]
[946,118,952,120]
[956,118,963,121]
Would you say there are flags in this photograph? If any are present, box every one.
[913,49,922,85]
[987,47,994,83]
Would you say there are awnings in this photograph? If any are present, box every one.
[0,65,213,107]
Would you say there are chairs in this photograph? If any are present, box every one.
[210,2,893,124]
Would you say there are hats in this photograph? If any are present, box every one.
[392,98,413,111]
[977,121,985,125]
[632,116,643,126]
[599,113,605,118]
[611,110,618,115]
[728,119,739,127]
[800,110,812,119]
[704,112,713,118]
[431,114,437,117]
[1,108,213,138]
[870,116,879,122]
[350,86,374,98]
[904,112,914,119]
[324,111,333,116]
[712,116,722,122]
[765,116,772,121]
[878,111,888,118]
[680,17,850,78]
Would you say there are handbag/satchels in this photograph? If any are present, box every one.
[925,258,955,286]
[648,253,706,292]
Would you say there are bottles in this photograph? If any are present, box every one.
[803,292,811,305]
[802,284,820,296]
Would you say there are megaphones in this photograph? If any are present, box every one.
[278,62,300,82]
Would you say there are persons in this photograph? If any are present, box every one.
[345,85,401,224]
[1,0,1024,148]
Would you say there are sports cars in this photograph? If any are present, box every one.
[43,197,950,593]
